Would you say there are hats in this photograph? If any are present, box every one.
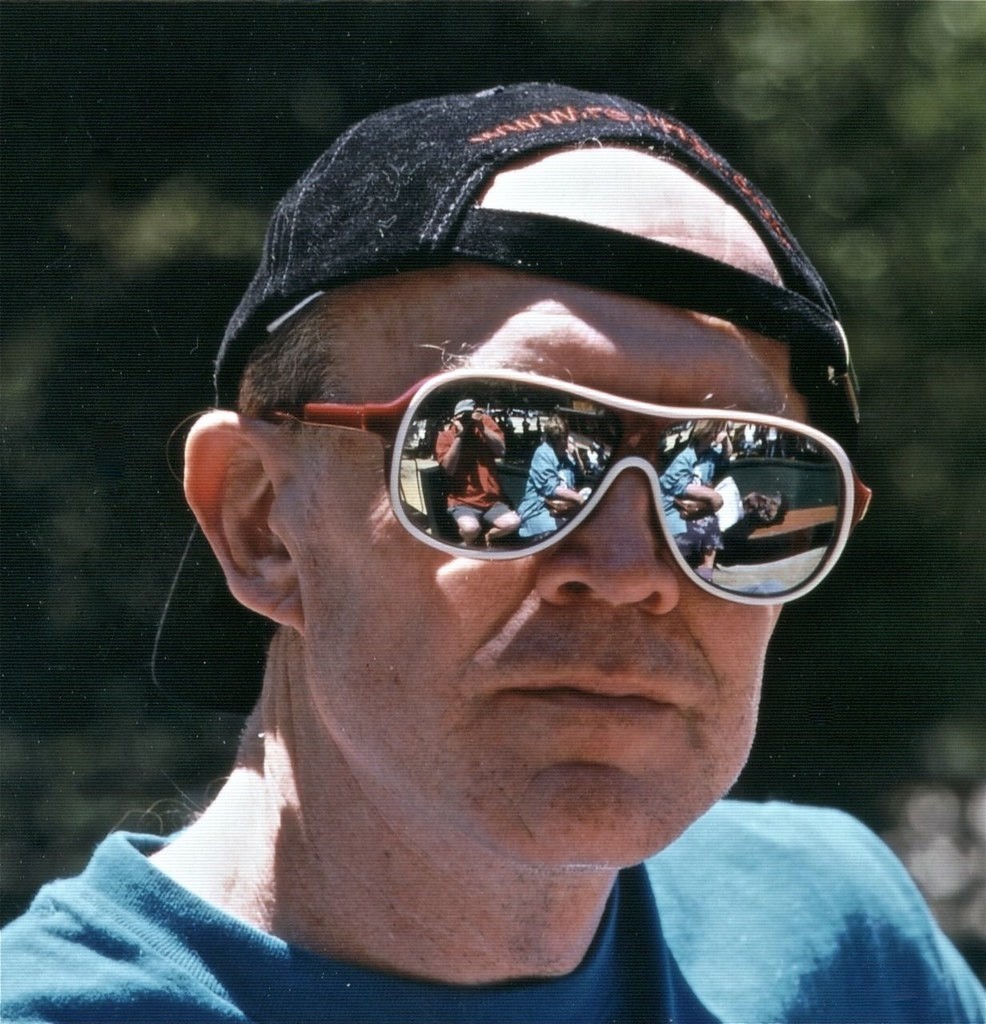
[454,399,475,416]
[147,79,863,714]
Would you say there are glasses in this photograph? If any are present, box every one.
[252,368,872,605]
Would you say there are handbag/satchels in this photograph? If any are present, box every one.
[674,493,714,520]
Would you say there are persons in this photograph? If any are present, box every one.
[435,399,521,550]
[517,413,586,545]
[0,82,986,1024]
[743,423,756,458]
[763,424,782,458]
[657,419,733,582]
